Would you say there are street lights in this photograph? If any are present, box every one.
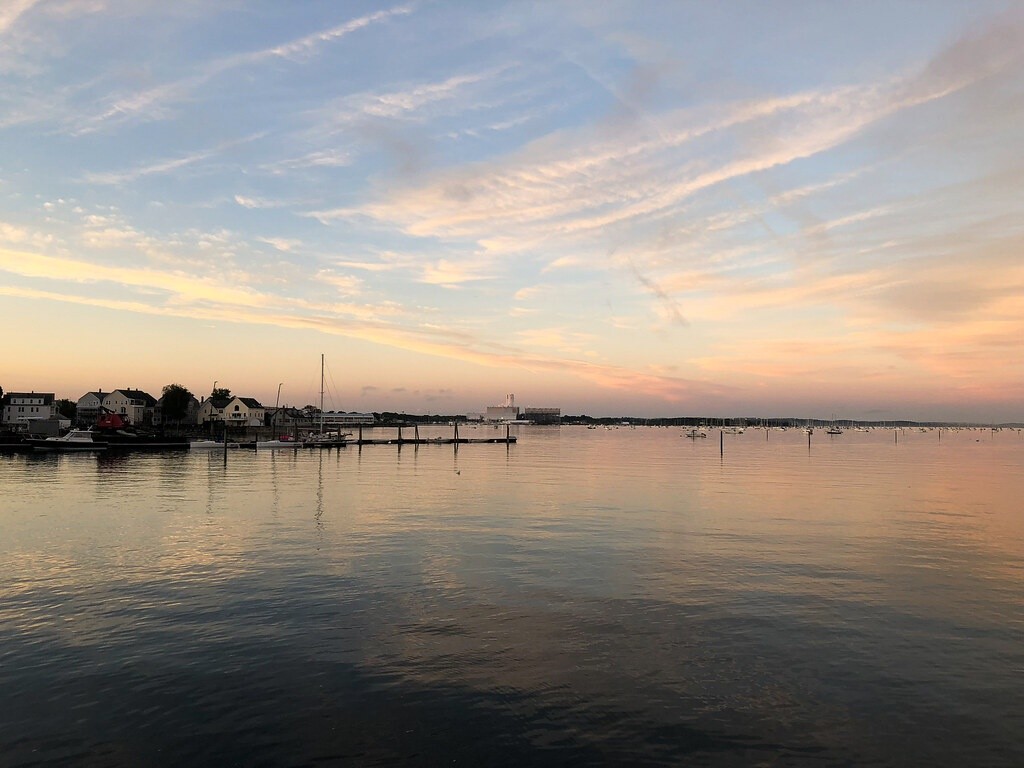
[210,380,218,436]
[274,382,283,424]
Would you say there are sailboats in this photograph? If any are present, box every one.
[302,354,352,447]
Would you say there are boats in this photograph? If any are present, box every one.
[190,440,258,448]
[257,440,303,448]
[26,405,190,451]
